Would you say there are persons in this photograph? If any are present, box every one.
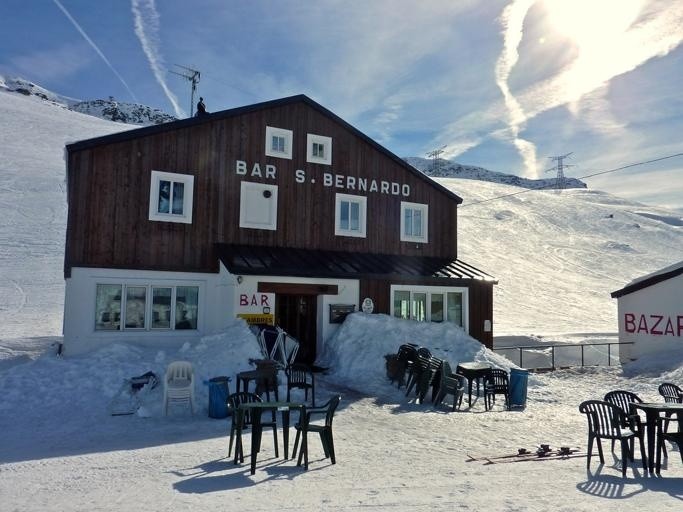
[197,97,209,116]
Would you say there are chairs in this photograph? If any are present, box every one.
[224,357,341,477]
[579,381,682,476]
[159,361,198,418]
[384,343,511,412]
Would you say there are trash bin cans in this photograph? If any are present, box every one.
[509,367,528,409]
[203,376,231,419]
